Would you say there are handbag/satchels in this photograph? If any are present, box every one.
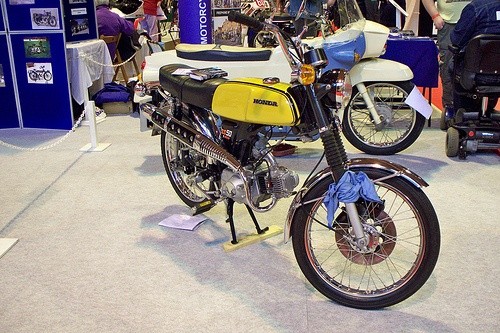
[127,80,163,115]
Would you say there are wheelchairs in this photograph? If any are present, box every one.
[445,34,500,158]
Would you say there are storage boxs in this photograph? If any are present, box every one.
[102,100,133,114]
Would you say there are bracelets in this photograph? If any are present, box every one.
[431,14,439,20]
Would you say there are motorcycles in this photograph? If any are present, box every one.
[131,8,443,305]
[135,13,427,157]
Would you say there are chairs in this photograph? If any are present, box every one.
[100,33,140,86]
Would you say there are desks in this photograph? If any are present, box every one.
[64,38,115,128]
[375,36,440,127]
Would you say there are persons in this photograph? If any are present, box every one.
[286,0,341,40]
[142,0,162,43]
[420,0,500,123]
[94,0,134,60]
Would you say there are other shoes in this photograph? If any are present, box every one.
[80,108,106,127]
